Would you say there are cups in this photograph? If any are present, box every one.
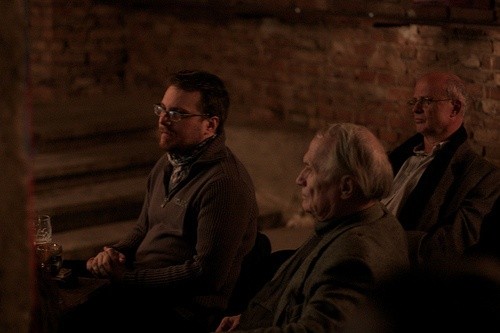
[34,215,51,244]
[36,243,62,276]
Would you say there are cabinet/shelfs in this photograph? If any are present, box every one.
[1,60,168,333]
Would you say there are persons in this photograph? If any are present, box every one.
[214,121,409,332]
[389,67,500,267]
[28,69,260,333]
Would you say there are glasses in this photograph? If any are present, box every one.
[153,104,212,122]
[408,96,455,106]
